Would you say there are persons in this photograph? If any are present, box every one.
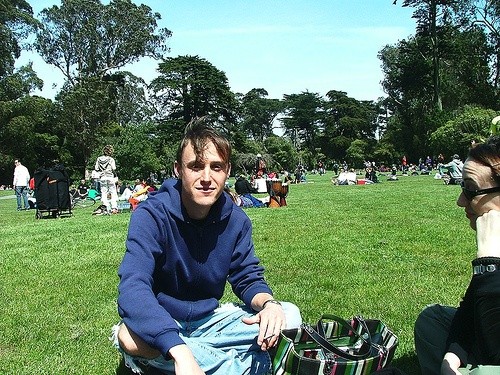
[28,179,158,211]
[414,139,500,375]
[111,115,302,375]
[95,145,119,214]
[235,153,464,195]
[13,158,30,211]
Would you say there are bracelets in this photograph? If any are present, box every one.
[262,300,281,308]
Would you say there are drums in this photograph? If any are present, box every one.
[279,183,289,206]
[266,178,281,208]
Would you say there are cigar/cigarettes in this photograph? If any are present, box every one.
[264,334,275,341]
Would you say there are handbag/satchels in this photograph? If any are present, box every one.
[272,313,399,375]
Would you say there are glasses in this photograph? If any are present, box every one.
[460,181,500,201]
